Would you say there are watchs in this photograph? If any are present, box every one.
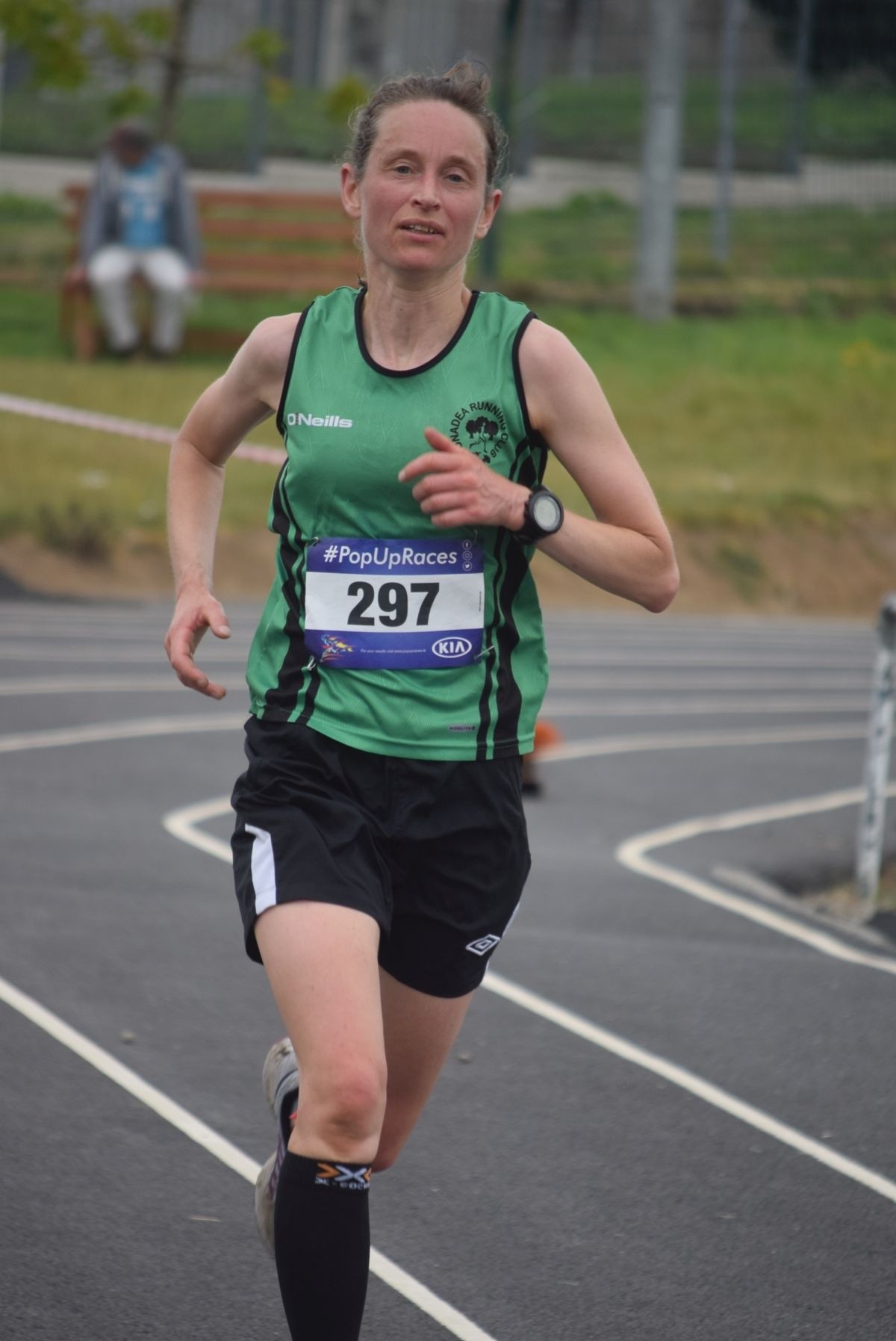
[509,481,564,545]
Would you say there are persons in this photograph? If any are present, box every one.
[162,55,681,1341]
[76,120,198,367]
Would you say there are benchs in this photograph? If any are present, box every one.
[57,179,366,358]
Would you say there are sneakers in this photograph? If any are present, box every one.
[253,1036,299,1261]
